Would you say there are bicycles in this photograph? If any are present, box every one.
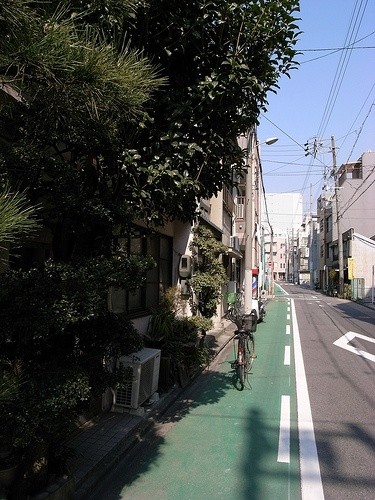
[226,306,259,391]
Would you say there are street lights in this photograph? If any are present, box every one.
[252,136,279,270]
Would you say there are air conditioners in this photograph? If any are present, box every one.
[114,348,160,407]
